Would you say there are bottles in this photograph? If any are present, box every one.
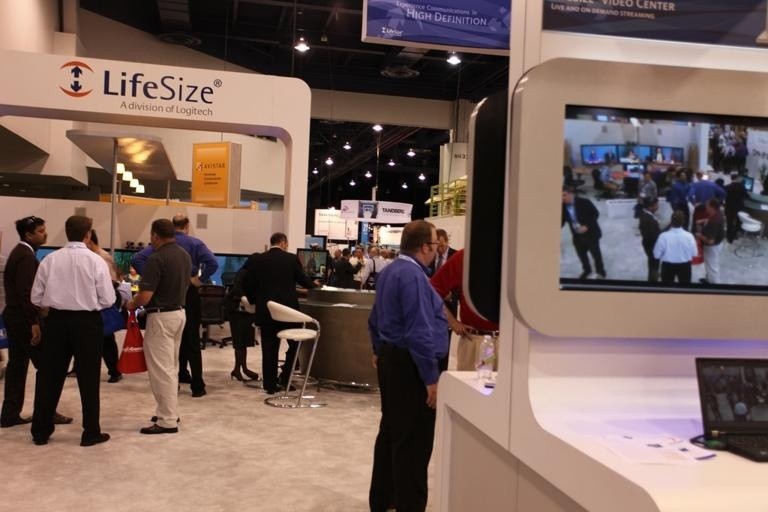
[477,335,495,385]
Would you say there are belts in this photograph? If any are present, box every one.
[466,328,500,338]
[148,306,185,312]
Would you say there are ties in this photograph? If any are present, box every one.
[437,254,443,269]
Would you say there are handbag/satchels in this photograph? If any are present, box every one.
[366,271,378,290]
[231,295,256,314]
[98,307,124,336]
[118,325,147,373]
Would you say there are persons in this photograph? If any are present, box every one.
[130,213,218,397]
[367,219,450,511]
[227,231,327,396]
[430,249,499,372]
[0,214,73,429]
[562,124,767,285]
[701,367,767,423]
[323,244,404,292]
[426,229,460,371]
[125,218,193,435]
[66,226,146,385]
[28,215,117,448]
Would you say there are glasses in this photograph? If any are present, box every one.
[26,215,38,228]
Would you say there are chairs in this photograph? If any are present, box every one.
[267,300,327,409]
[195,284,226,350]
[242,296,263,390]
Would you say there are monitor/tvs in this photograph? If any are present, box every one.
[202,253,252,287]
[102,249,140,275]
[508,58,768,341]
[581,144,617,165]
[741,175,754,192]
[652,145,683,166]
[35,246,62,263]
[297,248,329,280]
[462,92,507,322]
[617,144,652,164]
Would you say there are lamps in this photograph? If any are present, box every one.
[324,157,334,165]
[418,171,426,180]
[407,148,417,157]
[387,159,396,166]
[122,154,132,181]
[402,182,409,190]
[365,171,372,178]
[446,51,462,65]
[343,141,352,150]
[293,8,310,53]
[372,124,384,132]
[136,176,145,193]
[117,146,125,174]
[313,167,320,175]
[130,162,138,187]
[350,179,356,186]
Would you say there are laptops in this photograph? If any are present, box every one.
[695,358,768,461]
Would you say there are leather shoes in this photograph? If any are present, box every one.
[0,368,296,446]
